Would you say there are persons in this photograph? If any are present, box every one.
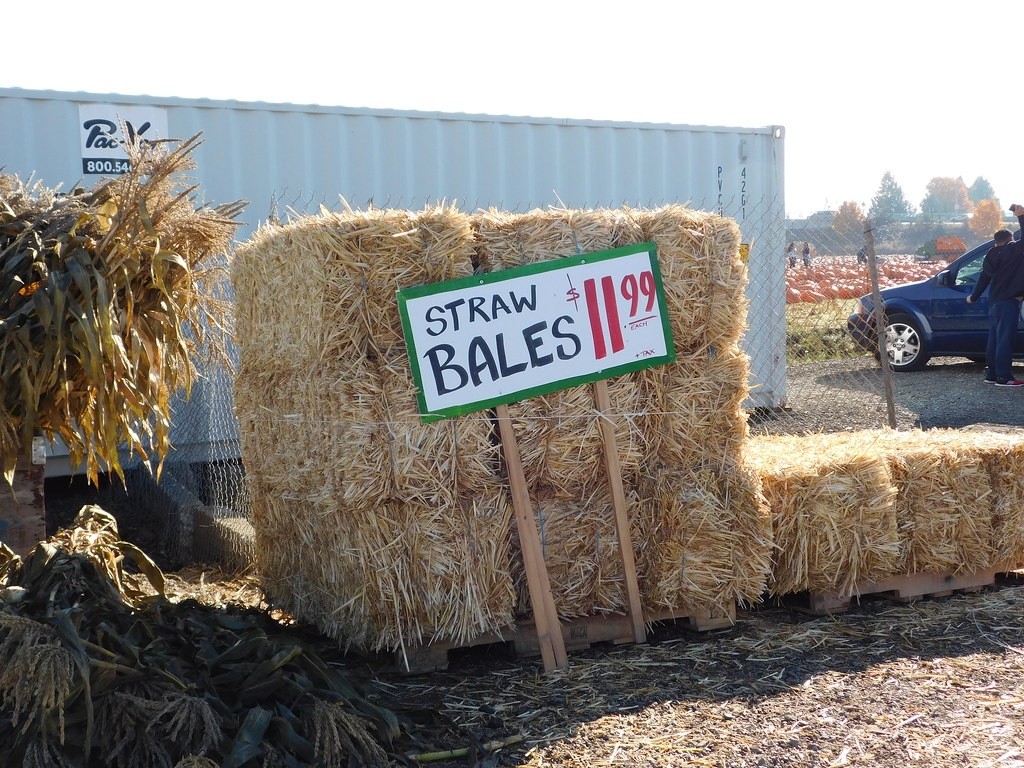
[965,204,1024,386]
[857,243,868,263]
[801,241,810,266]
[787,242,796,268]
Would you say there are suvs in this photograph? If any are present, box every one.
[846,235,1023,371]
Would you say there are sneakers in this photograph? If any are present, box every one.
[984,375,997,384]
[994,376,1024,387]
